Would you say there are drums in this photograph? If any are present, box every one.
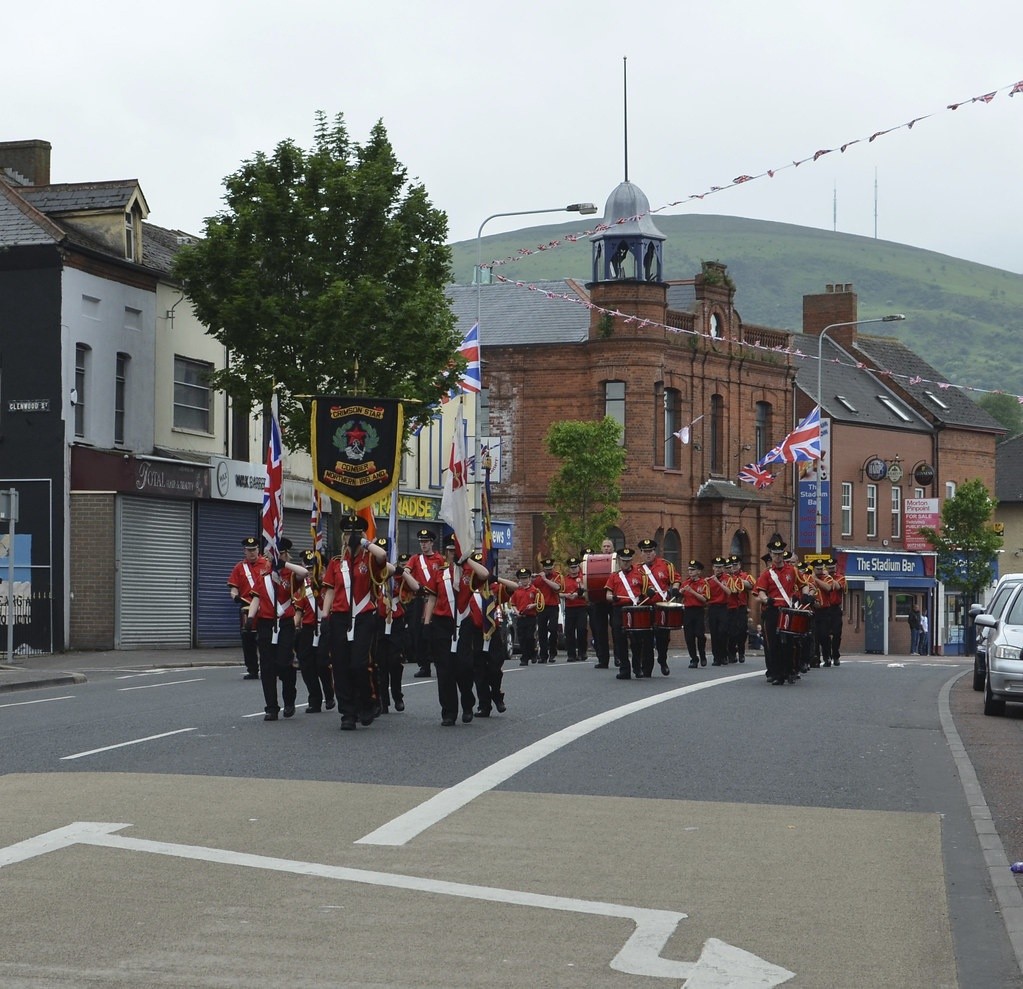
[776,606,813,636]
[582,552,620,604]
[621,605,654,633]
[240,605,260,633]
[656,602,685,631]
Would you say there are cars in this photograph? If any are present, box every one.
[975,582,1023,716]
[968,573,1023,691]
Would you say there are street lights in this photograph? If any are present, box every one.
[816,314,907,555]
[475,203,596,559]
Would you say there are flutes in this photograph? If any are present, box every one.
[816,573,825,576]
[707,571,725,581]
[689,577,695,585]
[829,571,838,574]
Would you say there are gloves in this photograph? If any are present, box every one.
[489,574,497,583]
[453,555,470,565]
[348,534,364,548]
[272,557,285,570]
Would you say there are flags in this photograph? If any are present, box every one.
[674,416,701,444]
[387,482,400,593]
[734,404,821,490]
[440,402,475,591]
[404,323,482,437]
[259,393,283,584]
[481,452,495,578]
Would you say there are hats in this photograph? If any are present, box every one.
[516,547,595,579]
[760,532,838,574]
[616,548,635,561]
[339,514,369,536]
[375,529,483,564]
[687,555,742,569]
[241,537,330,570]
[637,539,657,551]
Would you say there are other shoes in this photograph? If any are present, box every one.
[688,654,746,668]
[766,658,844,685]
[490,691,507,713]
[474,704,492,717]
[910,652,921,656]
[519,654,670,678]
[462,710,474,723]
[244,673,259,679]
[441,718,456,726]
[414,669,431,676]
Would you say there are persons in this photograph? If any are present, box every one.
[908,603,928,657]
[228,514,849,729]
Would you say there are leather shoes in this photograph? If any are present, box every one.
[264,696,406,730]
